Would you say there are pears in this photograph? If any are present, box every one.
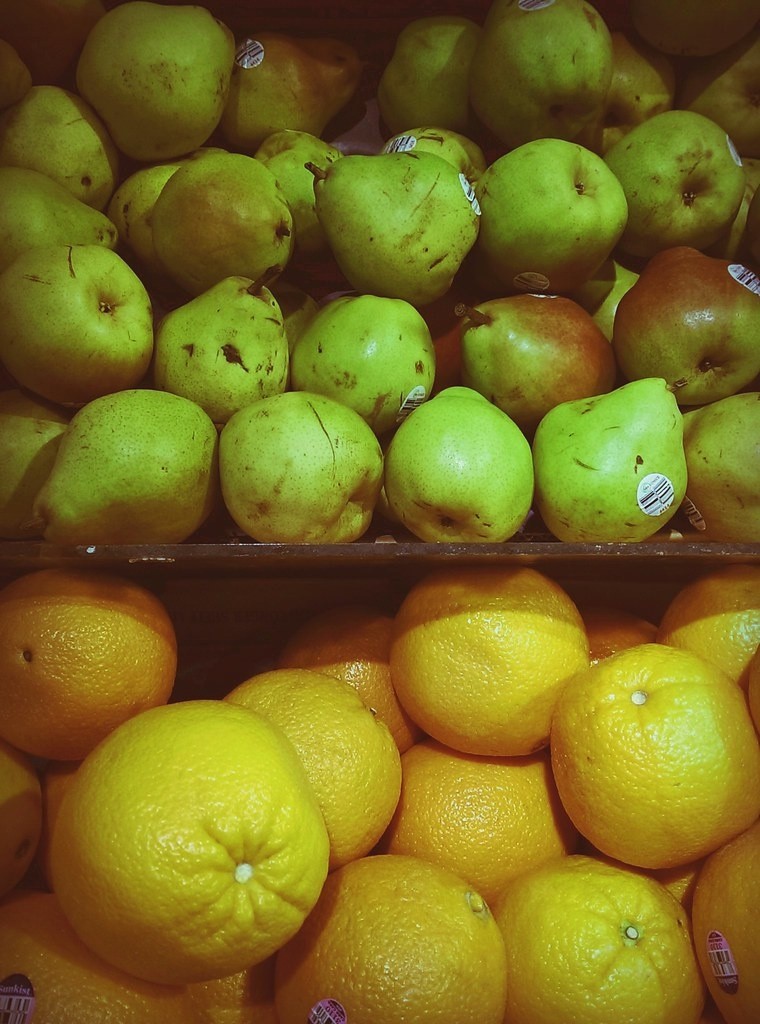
[0,0,760,548]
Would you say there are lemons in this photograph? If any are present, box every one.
[0,565,760,1024]
